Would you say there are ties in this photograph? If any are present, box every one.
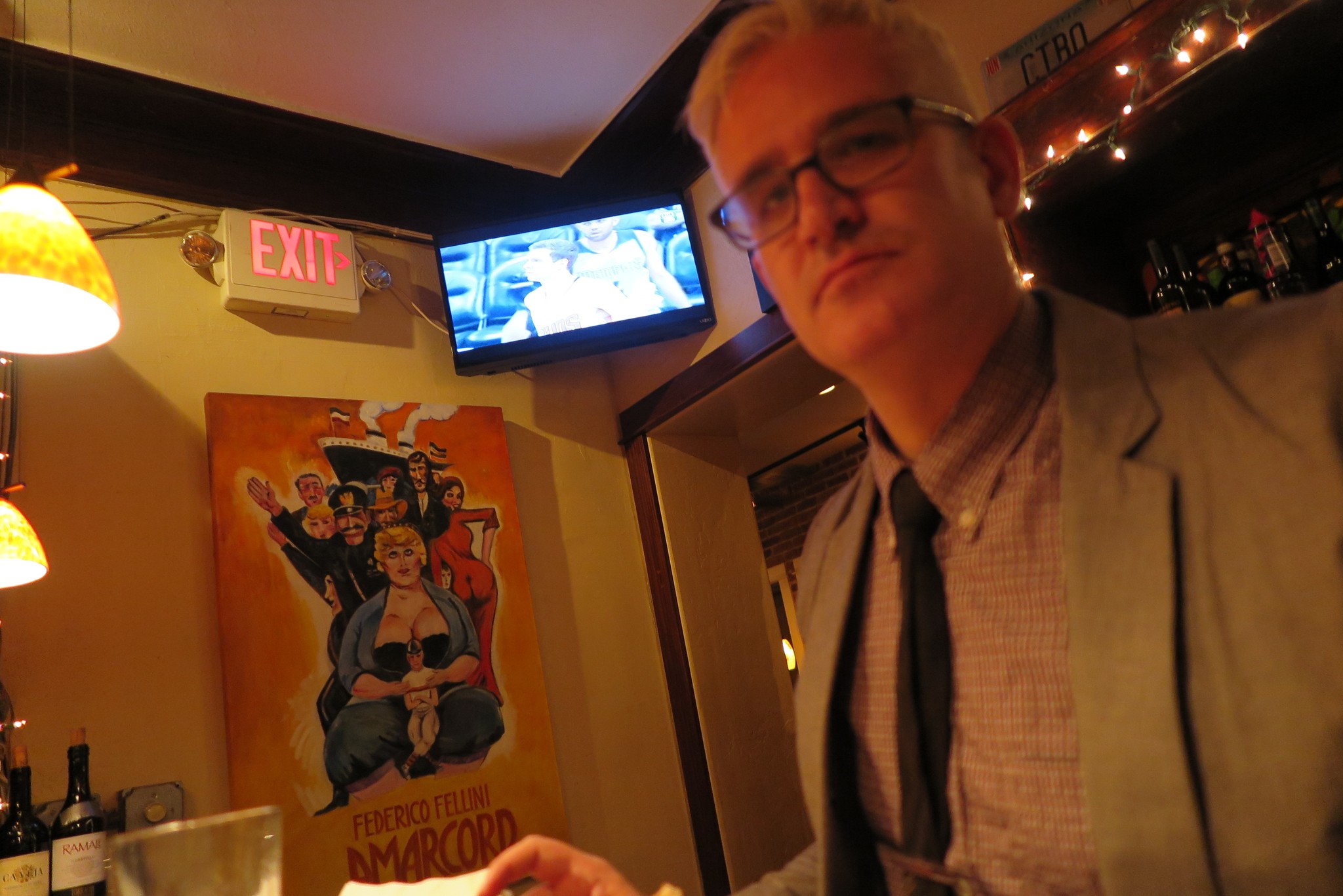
[888,468,954,896]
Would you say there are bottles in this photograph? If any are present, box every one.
[0,746,52,896]
[1145,178,1343,315]
[51,726,108,896]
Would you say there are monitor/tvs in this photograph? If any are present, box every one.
[429,181,718,377]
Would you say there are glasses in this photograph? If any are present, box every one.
[707,93,976,255]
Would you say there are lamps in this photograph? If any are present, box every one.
[179,209,391,323]
[0,165,122,594]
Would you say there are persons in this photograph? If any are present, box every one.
[501,238,642,345]
[477,0,1343,896]
[573,216,690,315]
